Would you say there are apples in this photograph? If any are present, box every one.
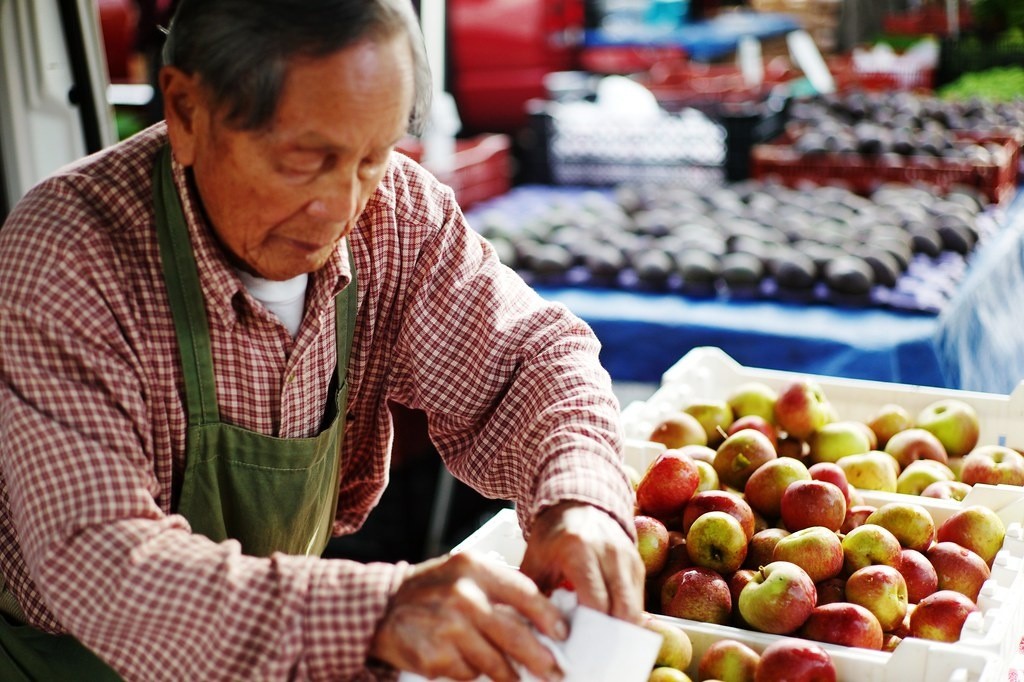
[627,383,1023,682]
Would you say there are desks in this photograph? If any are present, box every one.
[423,182,1024,572]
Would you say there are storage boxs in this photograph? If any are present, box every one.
[823,51,934,95]
[744,126,1019,204]
[401,344,1024,682]
[399,131,512,217]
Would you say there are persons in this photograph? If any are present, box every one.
[0,2,652,681]
